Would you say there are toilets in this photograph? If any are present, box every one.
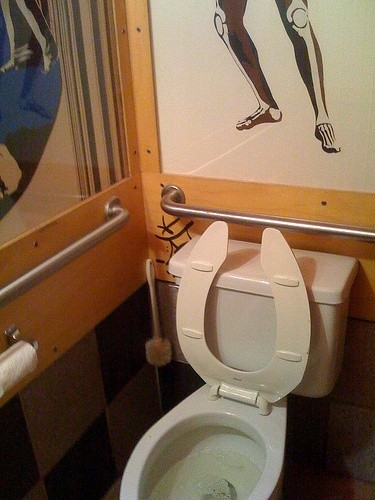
[119,220,359,499]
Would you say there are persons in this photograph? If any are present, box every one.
[214,0,341,156]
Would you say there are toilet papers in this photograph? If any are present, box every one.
[0,338,39,397]
